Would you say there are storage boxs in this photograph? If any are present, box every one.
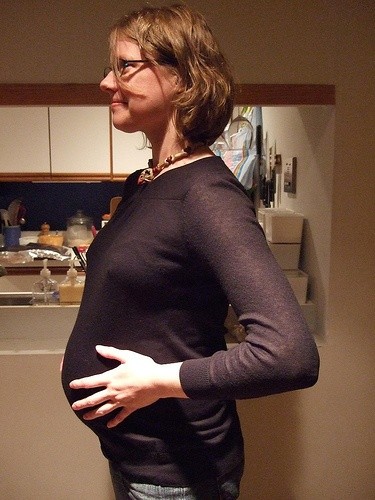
[255,207,309,304]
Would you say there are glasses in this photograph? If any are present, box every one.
[103,58,159,79]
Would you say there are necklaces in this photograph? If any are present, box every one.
[137,145,196,188]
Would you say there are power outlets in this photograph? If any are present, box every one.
[283,157,296,194]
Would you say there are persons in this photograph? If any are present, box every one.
[59,4,321,500]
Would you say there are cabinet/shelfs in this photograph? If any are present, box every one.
[0,103,153,184]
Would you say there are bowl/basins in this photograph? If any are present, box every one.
[19,237,38,245]
